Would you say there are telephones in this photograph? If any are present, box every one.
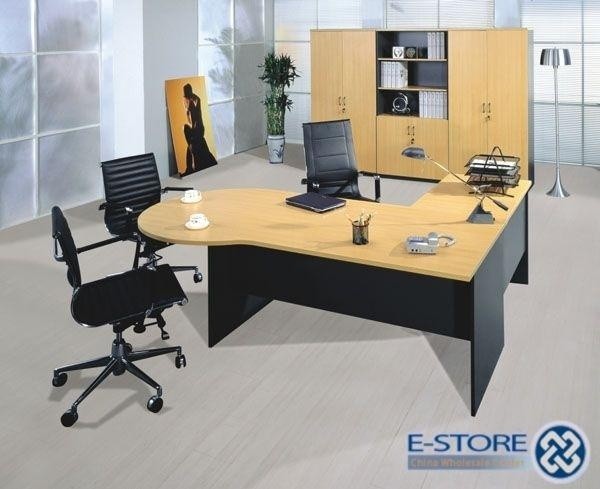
[405,230,440,256]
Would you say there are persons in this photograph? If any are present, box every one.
[182,83,206,174]
[183,123,218,168]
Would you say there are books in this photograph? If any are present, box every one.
[418,91,449,118]
[288,191,346,214]
[380,60,409,89]
[427,31,446,59]
[467,156,518,193]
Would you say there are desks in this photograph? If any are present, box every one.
[136,172,532,415]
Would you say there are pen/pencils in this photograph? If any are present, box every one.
[348,209,371,244]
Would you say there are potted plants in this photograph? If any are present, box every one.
[259,54,301,164]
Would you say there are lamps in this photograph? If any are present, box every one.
[540,48,573,198]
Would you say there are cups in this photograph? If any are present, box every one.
[352,219,370,245]
[181,188,210,231]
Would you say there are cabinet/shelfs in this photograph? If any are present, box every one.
[448,27,535,186]
[376,28,448,182]
[310,28,376,178]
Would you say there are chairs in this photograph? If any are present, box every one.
[301,119,381,201]
[99,153,202,340]
[50,206,187,427]
